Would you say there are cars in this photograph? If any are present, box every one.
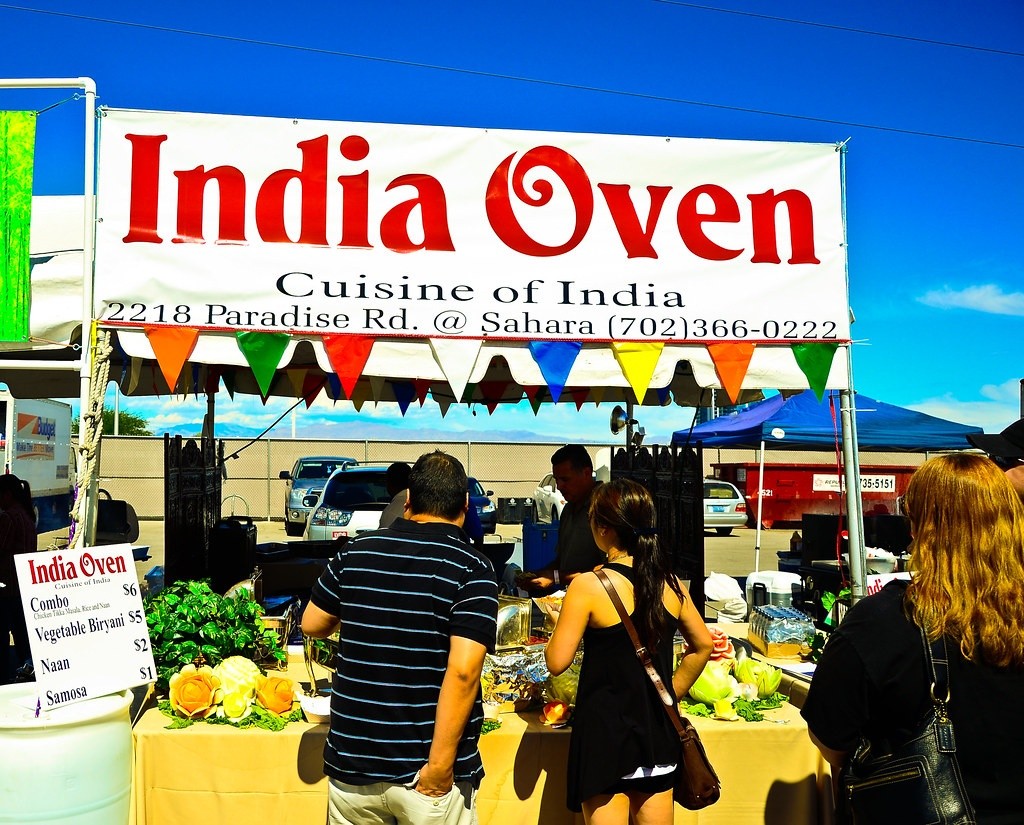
[704,477,748,536]
[279,456,361,535]
[466,476,498,534]
[302,460,416,541]
[533,472,568,523]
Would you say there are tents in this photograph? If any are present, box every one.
[671,385,984,577]
[0,193,864,616]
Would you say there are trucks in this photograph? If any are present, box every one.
[0,388,78,531]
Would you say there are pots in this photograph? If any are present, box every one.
[746,571,801,617]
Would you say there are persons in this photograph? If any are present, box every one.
[544,481,713,825]
[798,455,1024,825]
[513,445,608,590]
[0,474,37,668]
[302,449,498,825]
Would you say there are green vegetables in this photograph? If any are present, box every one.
[158,701,303,730]
[682,692,789,721]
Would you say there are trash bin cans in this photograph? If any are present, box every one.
[498,497,533,523]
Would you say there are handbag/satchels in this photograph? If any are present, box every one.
[844,698,976,825]
[95,488,139,542]
[673,716,721,810]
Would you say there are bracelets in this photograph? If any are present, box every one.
[553,570,560,584]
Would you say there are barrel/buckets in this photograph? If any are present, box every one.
[0,684,134,825]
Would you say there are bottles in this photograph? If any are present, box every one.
[749,605,816,644]
[814,633,823,662]
[790,531,802,551]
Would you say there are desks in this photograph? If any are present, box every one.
[128,653,834,825]
[707,621,831,709]
